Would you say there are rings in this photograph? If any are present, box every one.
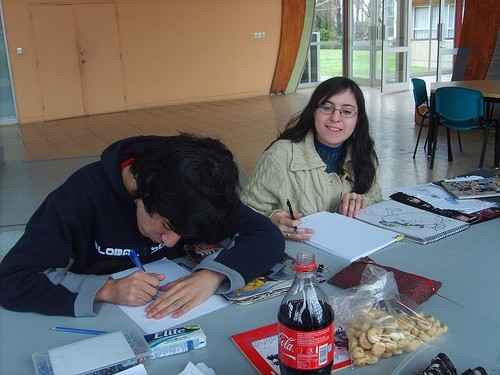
[351,197,357,201]
[288,233,291,239]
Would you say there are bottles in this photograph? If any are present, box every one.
[277,252,335,375]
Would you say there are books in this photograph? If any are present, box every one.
[350,200,472,245]
[442,174,500,201]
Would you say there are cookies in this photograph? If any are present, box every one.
[346,307,447,366]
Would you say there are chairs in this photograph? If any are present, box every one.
[411,74,499,168]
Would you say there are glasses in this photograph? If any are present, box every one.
[315,104,359,118]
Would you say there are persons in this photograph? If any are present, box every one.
[0,131,287,320]
[236,76,385,244]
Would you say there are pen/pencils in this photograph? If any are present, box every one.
[127,249,148,273]
[49,327,107,335]
[286,200,299,234]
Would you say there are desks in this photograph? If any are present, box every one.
[0,194,500,374]
[317,170,499,324]
[426,80,500,168]
